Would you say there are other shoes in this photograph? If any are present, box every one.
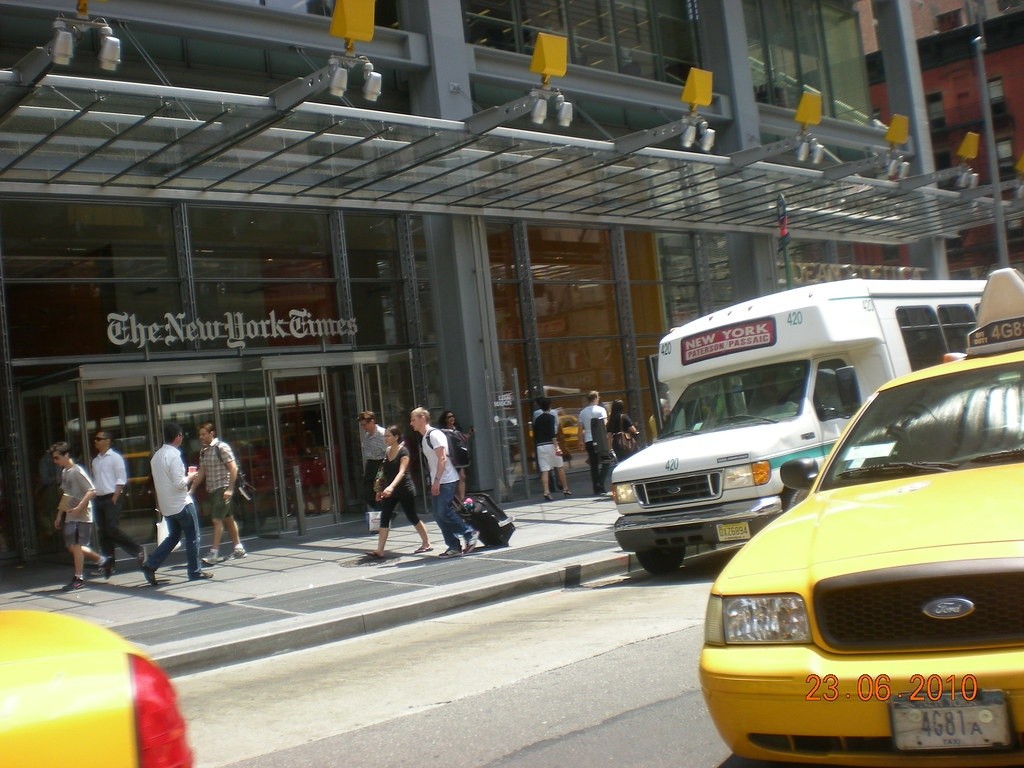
[550,486,556,493]
[557,484,564,491]
[594,484,608,494]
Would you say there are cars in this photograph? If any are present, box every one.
[185,429,326,527]
[528,414,584,456]
[698,346,1023,768]
[121,450,157,509]
[494,418,521,462]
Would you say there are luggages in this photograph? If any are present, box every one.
[451,493,516,546]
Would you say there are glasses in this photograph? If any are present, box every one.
[54,453,61,460]
[179,434,185,438]
[94,437,106,441]
[447,415,454,419]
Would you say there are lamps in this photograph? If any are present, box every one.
[820,156,910,181]
[957,132,979,164]
[897,166,979,190]
[329,0,374,54]
[1015,154,1024,176]
[726,133,824,168]
[794,91,822,133]
[607,115,716,154]
[885,114,909,152]
[265,55,382,112]
[680,67,713,115]
[960,180,1024,201]
[528,32,567,88]
[460,89,573,134]
[12,12,122,86]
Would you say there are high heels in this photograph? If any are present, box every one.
[543,493,553,502]
[563,489,572,497]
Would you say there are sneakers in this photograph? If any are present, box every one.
[100,557,112,579]
[462,530,479,554]
[201,554,218,565]
[223,548,248,561]
[91,567,116,575]
[136,546,147,568]
[62,575,84,592]
[141,565,158,586]
[188,571,213,581]
[438,545,462,559]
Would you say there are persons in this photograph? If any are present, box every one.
[439,410,475,500]
[607,399,639,461]
[410,407,480,558]
[189,423,247,565]
[533,396,573,500]
[47,441,114,591]
[356,411,398,534]
[365,425,434,558]
[577,390,610,495]
[140,423,214,586]
[91,431,147,578]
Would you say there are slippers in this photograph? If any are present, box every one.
[365,551,384,559]
[414,547,433,554]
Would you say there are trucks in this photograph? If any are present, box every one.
[609,275,987,578]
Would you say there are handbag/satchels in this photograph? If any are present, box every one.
[365,502,392,532]
[590,417,609,457]
[613,414,638,455]
[374,475,384,491]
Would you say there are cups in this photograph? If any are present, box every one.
[188,466,198,476]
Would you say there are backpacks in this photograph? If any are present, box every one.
[200,442,256,509]
[426,428,470,470]
